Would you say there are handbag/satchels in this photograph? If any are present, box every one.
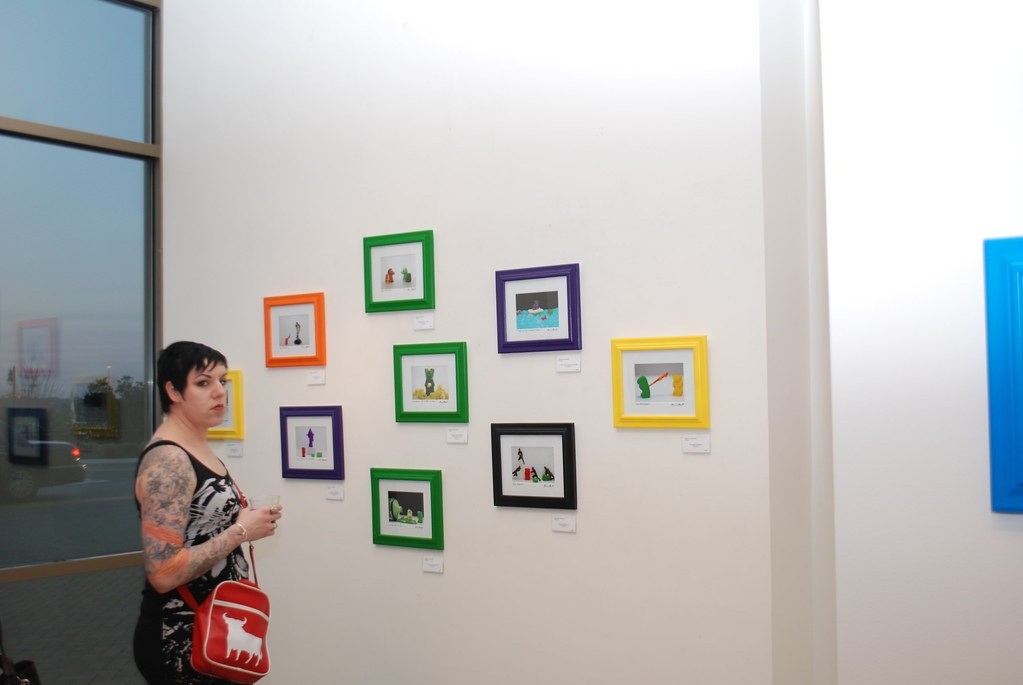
[190,580,270,684]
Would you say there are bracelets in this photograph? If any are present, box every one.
[236,522,248,541]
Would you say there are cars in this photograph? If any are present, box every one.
[0,396,89,502]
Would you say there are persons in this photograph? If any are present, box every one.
[134,340,285,685]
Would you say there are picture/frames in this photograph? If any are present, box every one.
[370,468,444,550]
[983,236,1023,515]
[495,263,581,353]
[393,342,468,423]
[363,230,435,313]
[16,318,56,376]
[279,406,345,481]
[490,423,577,510]
[610,335,711,430]
[6,407,49,466]
[263,293,326,368]
[72,376,121,438]
[204,370,244,440]
